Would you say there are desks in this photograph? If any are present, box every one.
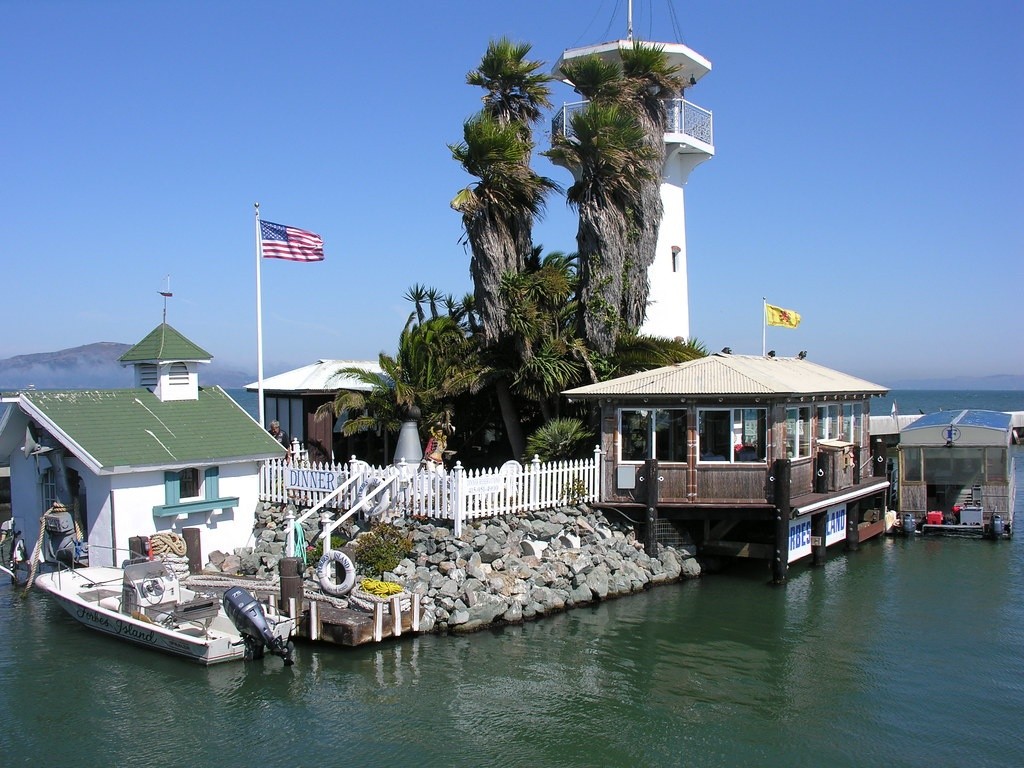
[738,451,758,461]
[703,455,726,461]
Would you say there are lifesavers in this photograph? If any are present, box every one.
[317,550,354,595]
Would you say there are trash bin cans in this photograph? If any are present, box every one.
[991,514,1004,534]
[902,512,916,532]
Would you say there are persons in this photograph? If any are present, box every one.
[267,419,290,481]
[421,427,448,478]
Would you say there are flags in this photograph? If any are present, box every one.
[260,219,324,262]
[766,302,801,329]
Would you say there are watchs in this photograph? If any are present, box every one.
[287,455,290,458]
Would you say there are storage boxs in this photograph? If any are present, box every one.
[927,512,944,524]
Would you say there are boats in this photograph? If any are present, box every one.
[35,510,296,668]
